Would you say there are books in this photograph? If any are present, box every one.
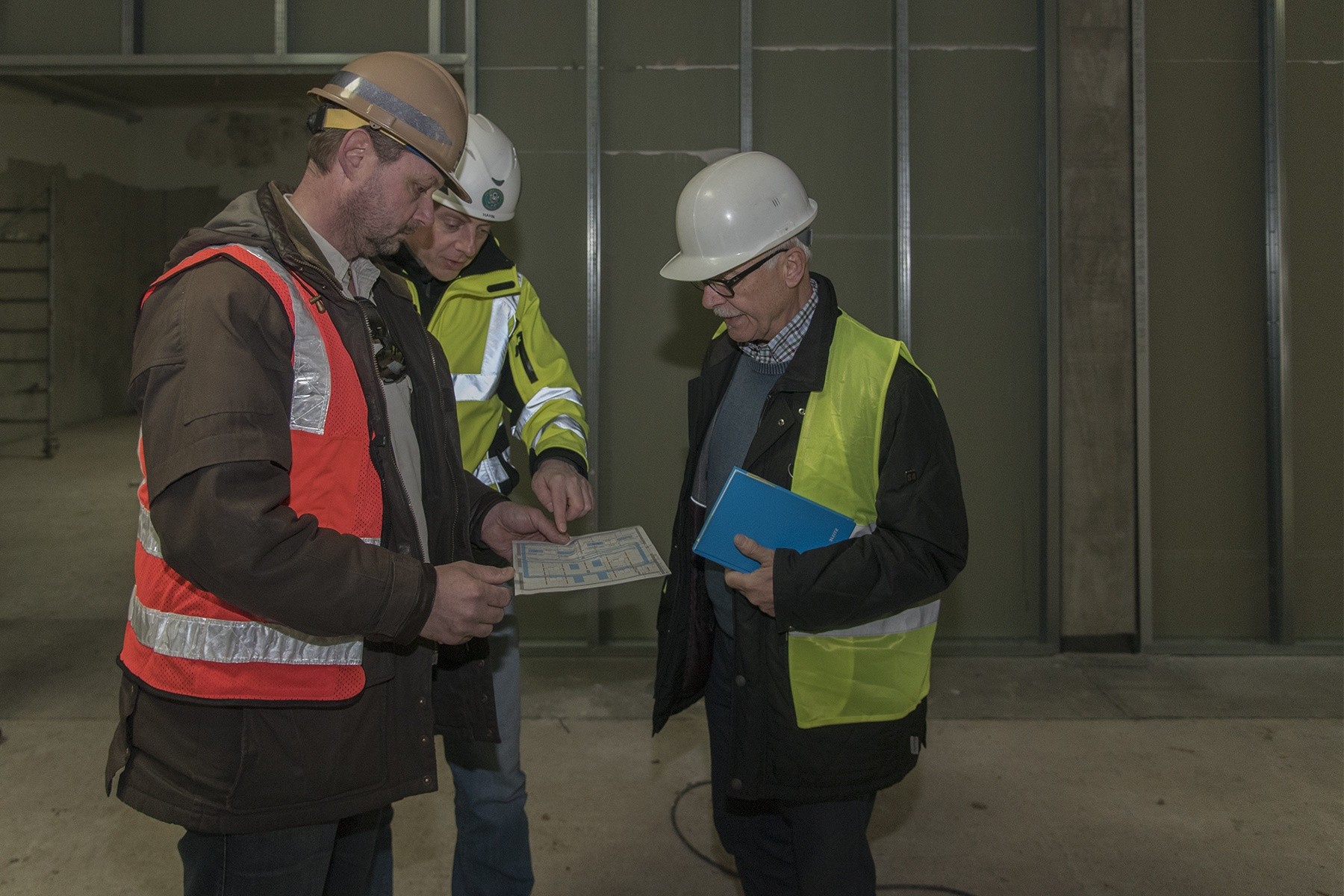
[693,467,857,576]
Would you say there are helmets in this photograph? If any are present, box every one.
[433,113,521,221]
[307,51,472,204]
[659,151,818,281]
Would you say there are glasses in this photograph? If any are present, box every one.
[689,249,789,297]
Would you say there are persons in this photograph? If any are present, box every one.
[106,48,595,896]
[653,151,968,896]
